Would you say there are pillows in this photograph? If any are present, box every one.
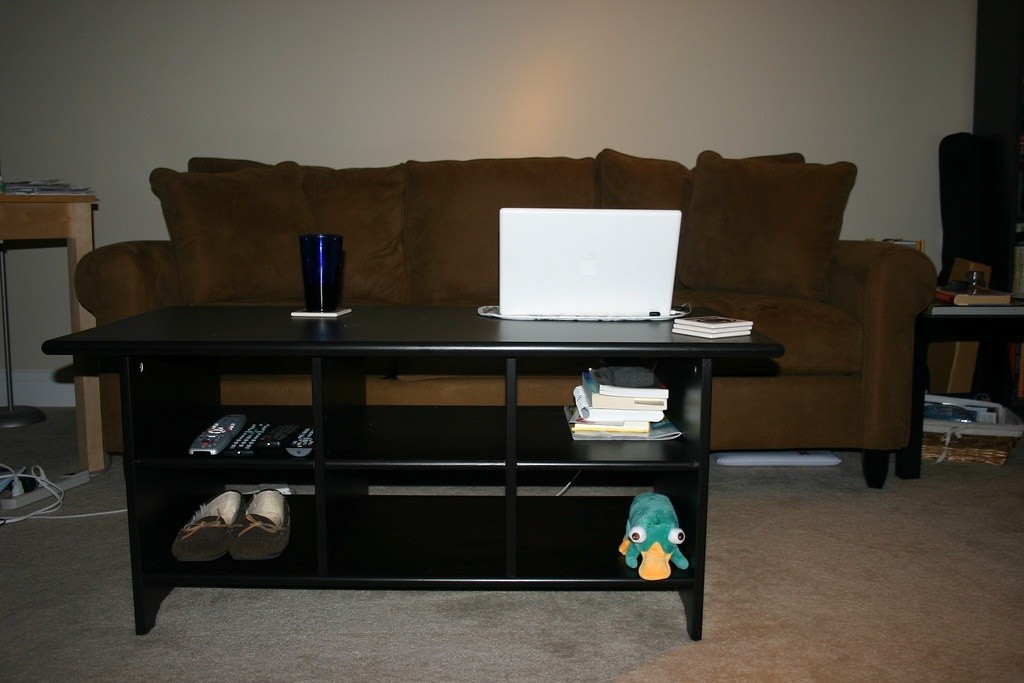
[151,154,858,304]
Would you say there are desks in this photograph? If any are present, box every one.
[2,193,104,471]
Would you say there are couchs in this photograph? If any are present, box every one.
[75,157,937,490]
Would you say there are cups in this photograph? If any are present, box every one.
[297,233,343,312]
[965,271,984,295]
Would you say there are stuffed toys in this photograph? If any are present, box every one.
[619,492,689,581]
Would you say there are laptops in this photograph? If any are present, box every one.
[499,207,681,318]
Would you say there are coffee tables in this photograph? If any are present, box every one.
[896,315,1024,480]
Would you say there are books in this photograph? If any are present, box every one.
[563,366,682,441]
[0,178,95,196]
[673,317,753,339]
[879,223,1024,308]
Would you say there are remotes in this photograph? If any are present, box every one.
[191,412,315,457]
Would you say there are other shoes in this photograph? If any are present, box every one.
[229,488,290,561]
[173,490,243,563]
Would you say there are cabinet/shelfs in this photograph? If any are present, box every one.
[41,306,784,641]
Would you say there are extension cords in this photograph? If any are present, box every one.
[0,470,93,511]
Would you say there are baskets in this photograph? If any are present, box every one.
[922,427,1014,466]
